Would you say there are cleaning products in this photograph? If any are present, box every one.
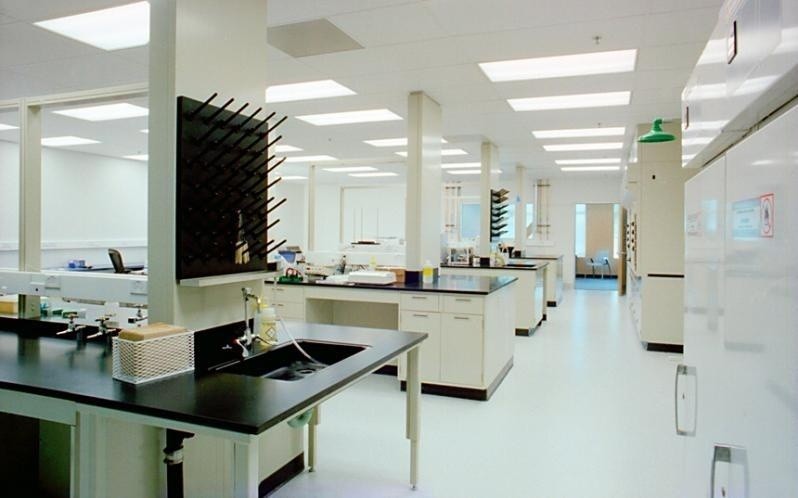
[253,277,278,350]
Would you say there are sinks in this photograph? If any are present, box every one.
[348,269,398,285]
[218,341,365,380]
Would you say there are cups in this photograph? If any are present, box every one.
[473,258,481,267]
[68,260,86,268]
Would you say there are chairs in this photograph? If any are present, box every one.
[108,248,132,273]
[585,248,609,280]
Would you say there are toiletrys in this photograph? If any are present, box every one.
[422,258,434,283]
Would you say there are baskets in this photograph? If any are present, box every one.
[111,330,195,386]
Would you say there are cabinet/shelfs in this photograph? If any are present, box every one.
[442,281,515,400]
[265,283,333,324]
[636,113,684,144]
[400,293,440,395]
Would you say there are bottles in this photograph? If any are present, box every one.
[423,260,433,283]
[335,255,347,272]
[253,304,279,346]
[369,255,377,272]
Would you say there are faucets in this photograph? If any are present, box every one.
[86,318,118,339]
[128,304,147,323]
[234,288,278,358]
[57,314,85,342]
